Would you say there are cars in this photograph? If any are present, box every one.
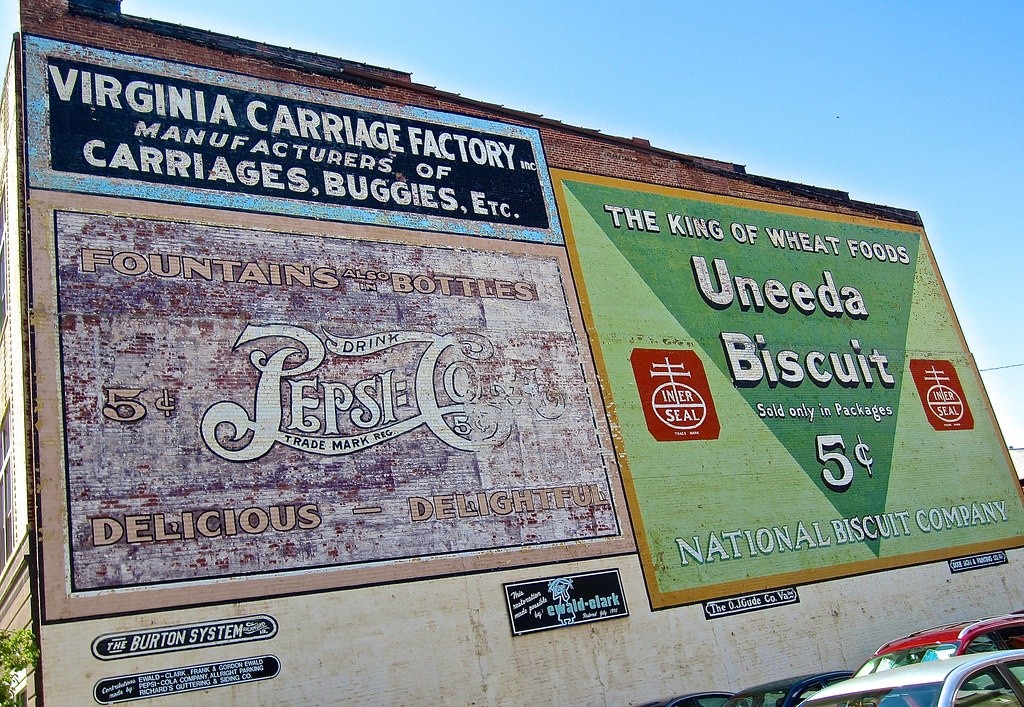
[794,650,1024,707]
[718,671,853,707]
[632,691,768,707]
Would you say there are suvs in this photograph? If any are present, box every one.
[842,611,1024,707]
